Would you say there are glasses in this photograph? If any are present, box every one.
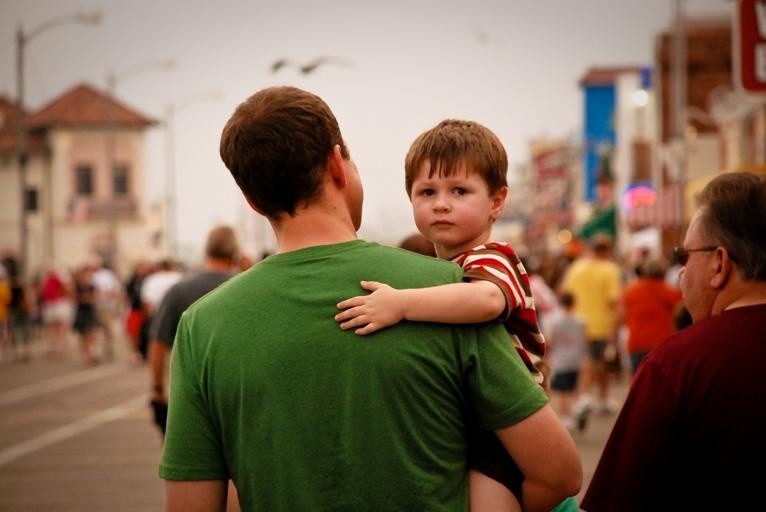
[670,244,740,268]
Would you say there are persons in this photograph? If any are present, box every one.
[581,172,765,512]
[397,235,437,256]
[515,231,691,442]
[158,84,584,512]
[0,252,191,368]
[336,115,546,512]
[149,226,273,440]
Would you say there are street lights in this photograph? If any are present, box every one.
[14,12,106,278]
